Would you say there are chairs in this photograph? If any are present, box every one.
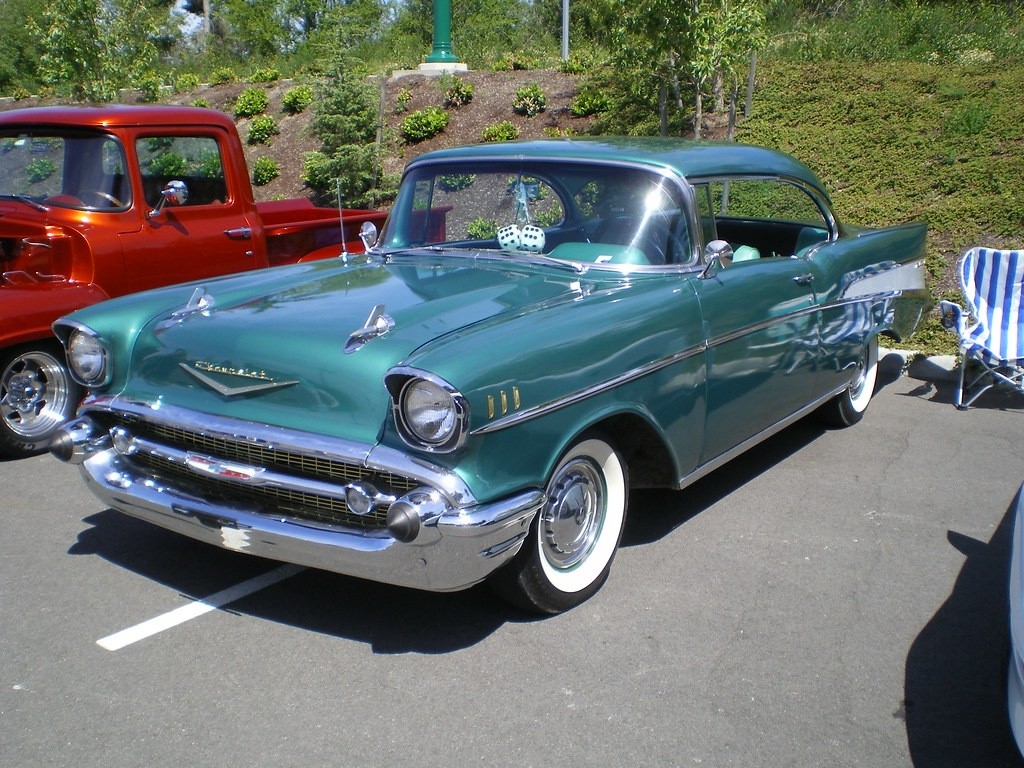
[940,246,1024,411]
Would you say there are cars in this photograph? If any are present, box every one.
[49,134,931,620]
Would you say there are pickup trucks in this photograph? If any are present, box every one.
[0,104,453,456]
